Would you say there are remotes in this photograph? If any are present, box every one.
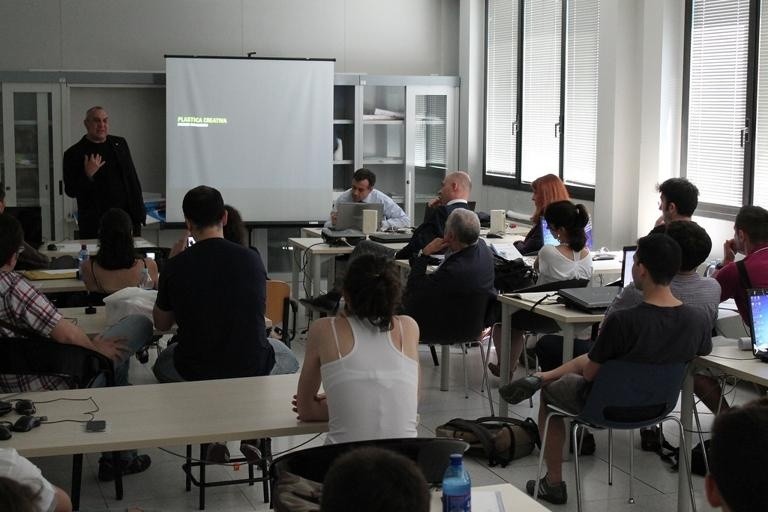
[592,254,613,261]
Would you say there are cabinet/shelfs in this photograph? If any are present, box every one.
[333,73,353,204]
[0,74,63,248]
[357,75,464,229]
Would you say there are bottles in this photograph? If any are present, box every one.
[441,451,473,512]
[78,243,89,276]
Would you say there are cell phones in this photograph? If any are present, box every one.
[87,420,106,432]
[146,252,155,261]
[187,236,197,248]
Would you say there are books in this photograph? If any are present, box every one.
[491,244,522,260]
[503,291,560,305]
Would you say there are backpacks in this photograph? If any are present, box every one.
[436,416,541,468]
[494,254,538,290]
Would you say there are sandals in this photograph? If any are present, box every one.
[136,345,149,364]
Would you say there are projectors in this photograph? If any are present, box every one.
[322,228,366,247]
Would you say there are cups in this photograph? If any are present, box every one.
[361,209,378,234]
[490,210,506,235]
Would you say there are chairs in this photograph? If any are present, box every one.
[1,211,767,512]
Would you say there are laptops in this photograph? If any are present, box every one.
[427,202,476,234]
[369,235,413,242]
[2,206,42,250]
[746,288,768,361]
[430,254,445,261]
[335,203,384,230]
[559,286,624,308]
[540,216,592,251]
[621,246,636,288]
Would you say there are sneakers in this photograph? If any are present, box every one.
[99,454,151,481]
[240,439,263,460]
[498,375,542,405]
[570,433,596,456]
[527,472,567,504]
[641,426,660,451]
[208,445,230,463]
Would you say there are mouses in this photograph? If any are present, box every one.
[487,233,500,238]
[0,399,41,441]
[48,244,57,250]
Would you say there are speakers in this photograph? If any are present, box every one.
[363,209,378,234]
[490,210,506,233]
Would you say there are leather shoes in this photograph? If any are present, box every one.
[488,362,513,380]
[299,295,337,312]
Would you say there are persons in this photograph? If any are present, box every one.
[0,216,151,482]
[292,255,419,445]
[321,447,431,512]
[500,234,712,504]
[152,186,297,466]
[694,207,768,413]
[535,221,721,451]
[403,208,495,342]
[0,447,72,512]
[656,178,699,225]
[59,102,146,236]
[300,171,472,314]
[80,209,159,363]
[326,168,411,284]
[488,201,592,379]
[704,398,768,512]
[513,174,569,253]
[223,205,267,280]
